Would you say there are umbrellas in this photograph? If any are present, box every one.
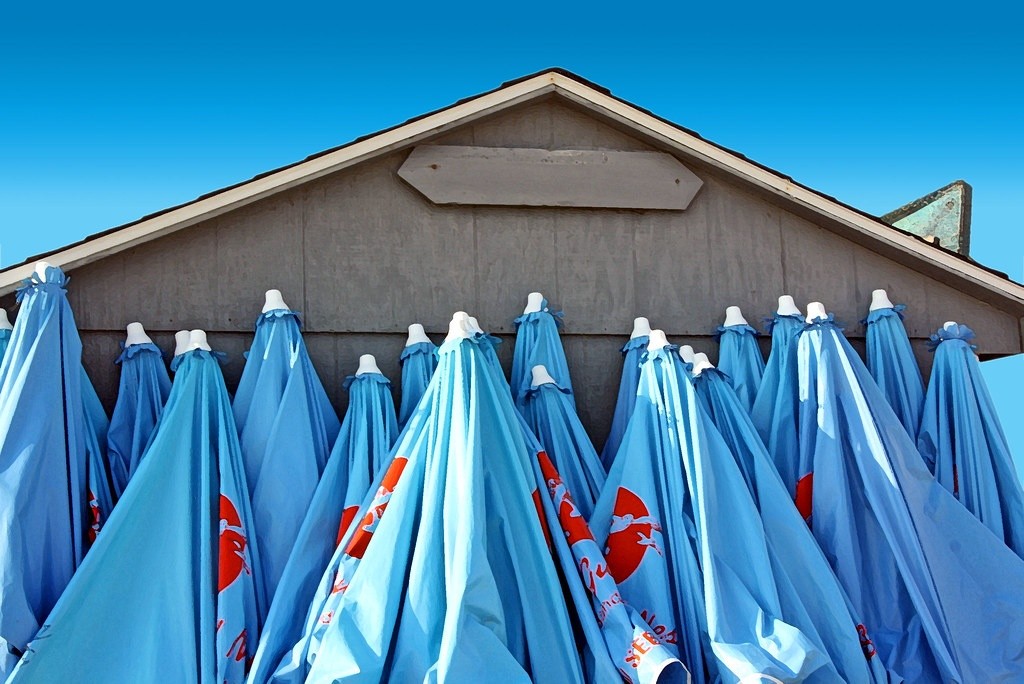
[0,261,1024,684]
